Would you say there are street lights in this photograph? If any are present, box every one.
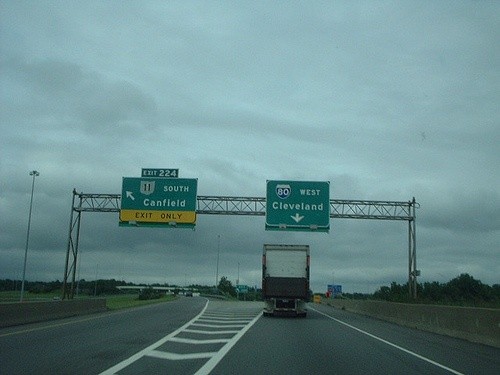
[19,170,40,301]
[215,235,220,287]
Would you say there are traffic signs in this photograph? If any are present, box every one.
[235,284,248,294]
[118,176,198,224]
[265,179,331,228]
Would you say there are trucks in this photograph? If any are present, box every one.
[261,244,311,318]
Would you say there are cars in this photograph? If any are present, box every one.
[186,290,192,297]
